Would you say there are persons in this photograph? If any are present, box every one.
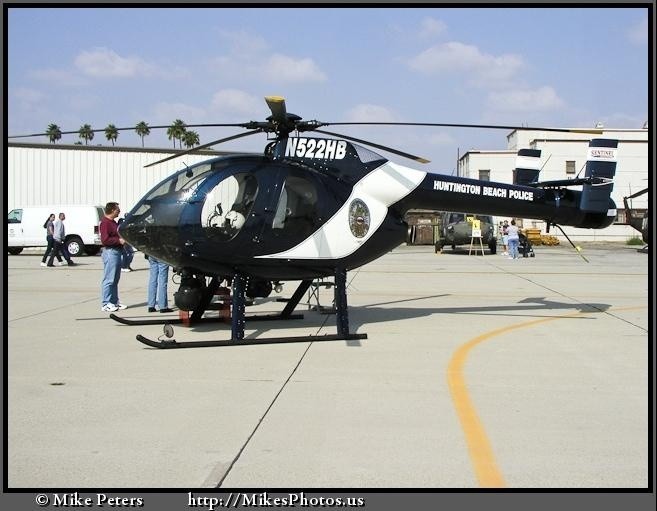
[503,219,518,260]
[39,213,65,267]
[99,201,128,312]
[147,256,173,313]
[119,213,134,272]
[46,213,78,266]
[499,220,508,256]
[499,223,504,239]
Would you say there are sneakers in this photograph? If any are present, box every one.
[121,268,131,273]
[115,304,128,310]
[101,305,118,312]
[39,260,77,267]
[148,307,173,313]
[500,252,518,260]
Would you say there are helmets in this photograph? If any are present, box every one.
[225,210,245,229]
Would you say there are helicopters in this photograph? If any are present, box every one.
[435,210,496,254]
[7,95,649,350]
[613,188,649,253]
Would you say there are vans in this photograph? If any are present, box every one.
[8,204,106,256]
